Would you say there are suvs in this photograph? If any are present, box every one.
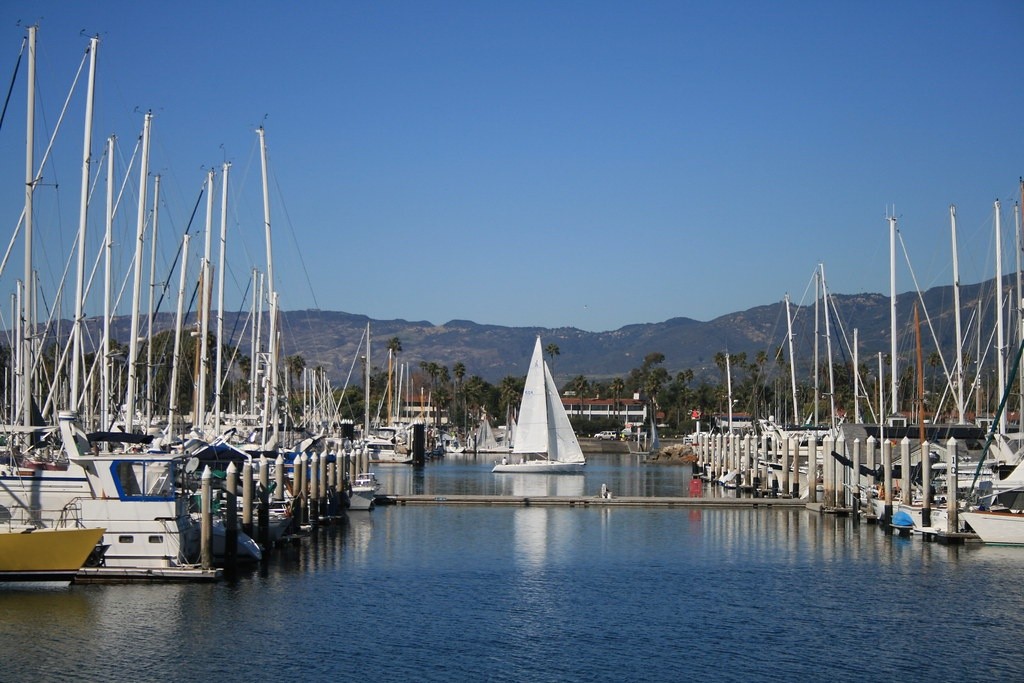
[594,430,617,441]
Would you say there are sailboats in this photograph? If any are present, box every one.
[466,403,517,454]
[686,177,1024,549]
[491,335,587,472]
[0,27,466,589]
